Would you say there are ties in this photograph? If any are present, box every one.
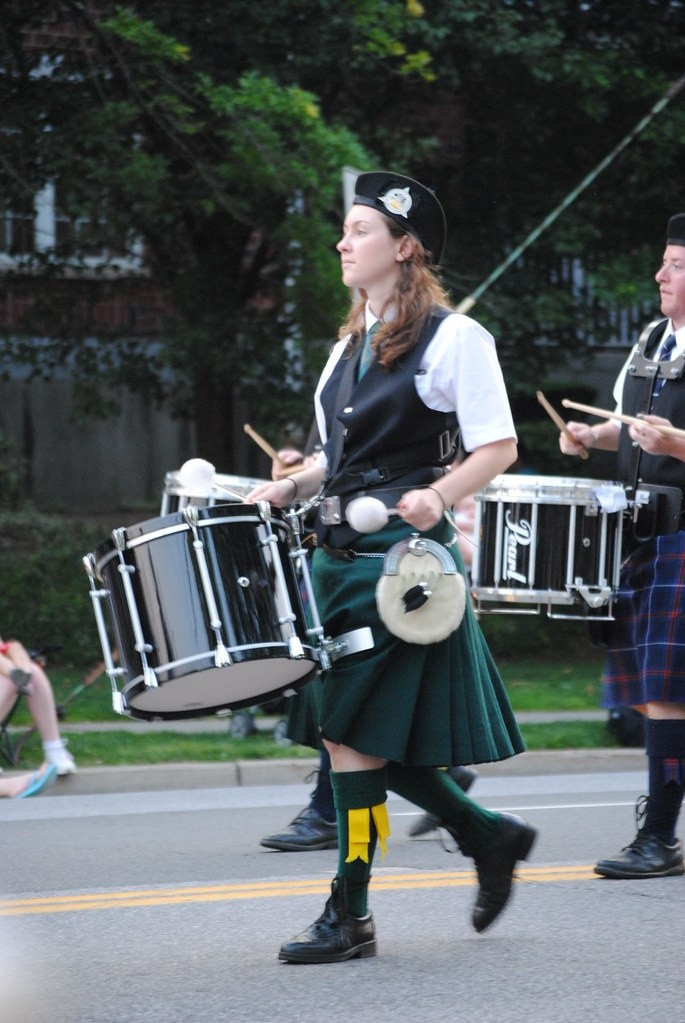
[649,334,677,413]
[357,321,382,384]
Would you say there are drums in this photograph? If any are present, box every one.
[82,500,332,724]
[159,469,304,519]
[470,473,625,623]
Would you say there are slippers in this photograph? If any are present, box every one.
[17,762,56,799]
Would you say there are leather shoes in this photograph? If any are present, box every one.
[260,808,339,850]
[408,766,478,837]
[594,829,685,878]
[462,811,538,932]
[280,876,376,963]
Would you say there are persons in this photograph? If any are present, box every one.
[559,212,685,879]
[0,636,77,799]
[241,172,541,962]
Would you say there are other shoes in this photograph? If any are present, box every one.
[43,738,77,775]
[11,668,33,687]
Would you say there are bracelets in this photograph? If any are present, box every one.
[281,478,298,500]
[427,487,446,512]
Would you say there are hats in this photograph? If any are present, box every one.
[666,212,685,247]
[354,171,446,269]
